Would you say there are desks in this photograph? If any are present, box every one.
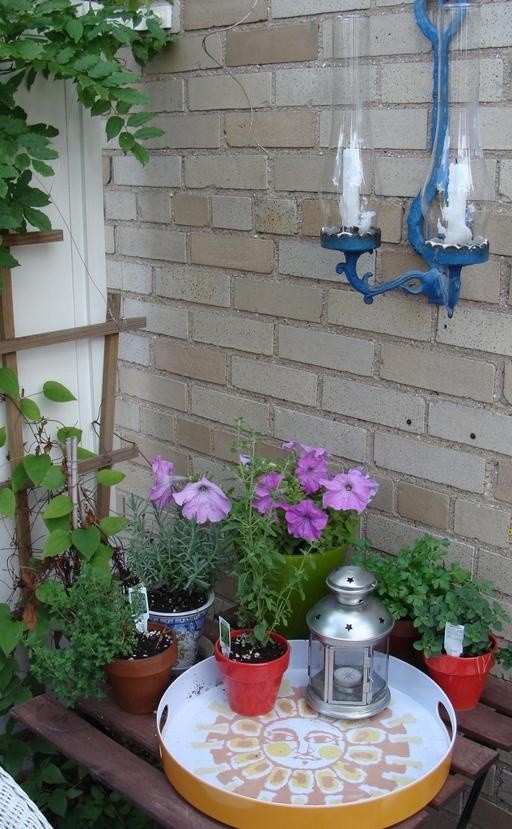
[6,573,512,828]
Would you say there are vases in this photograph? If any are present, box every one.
[150,580,216,676]
[231,536,351,638]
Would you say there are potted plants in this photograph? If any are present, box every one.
[210,413,323,718]
[354,528,512,711]
[24,568,178,716]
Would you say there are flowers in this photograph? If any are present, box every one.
[237,442,379,541]
[150,453,232,524]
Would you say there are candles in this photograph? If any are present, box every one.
[445,157,473,244]
[337,142,362,229]
